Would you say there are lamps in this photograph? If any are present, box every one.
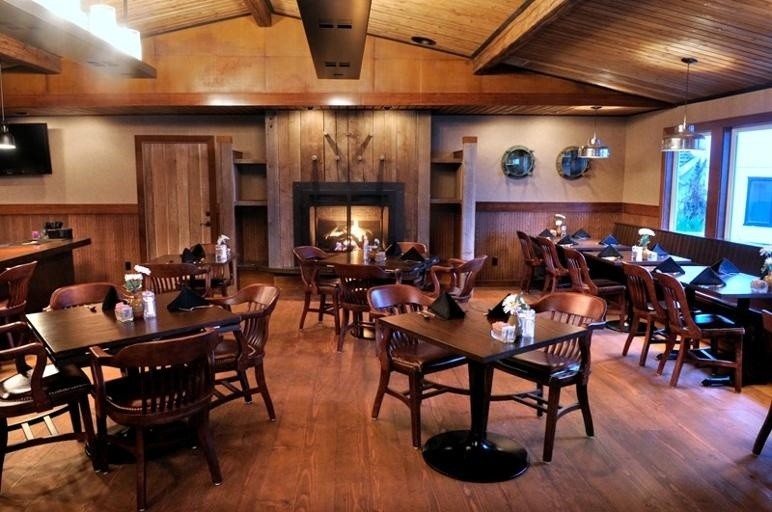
[661,59,708,152]
[578,104,611,159]
[0,64,17,150]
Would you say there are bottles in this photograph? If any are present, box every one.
[631,246,643,261]
[142,290,157,319]
[561,226,567,238]
[550,229,557,236]
[215,244,231,264]
[517,309,535,338]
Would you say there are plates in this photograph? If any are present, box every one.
[375,257,387,262]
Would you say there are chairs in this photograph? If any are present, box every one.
[0,230,772,512]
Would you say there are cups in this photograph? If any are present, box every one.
[647,251,657,261]
[201,243,215,263]
[376,252,386,260]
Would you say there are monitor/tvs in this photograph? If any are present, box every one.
[0,121,54,177]
